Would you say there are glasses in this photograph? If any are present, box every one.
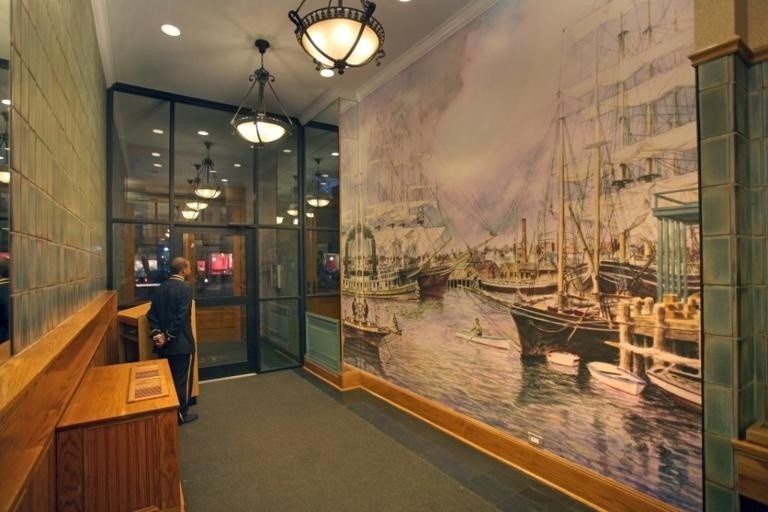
[288,0,387,77]
[276,157,331,224]
[181,142,223,220]
[230,38,294,150]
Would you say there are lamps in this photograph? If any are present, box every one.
[196,250,233,293]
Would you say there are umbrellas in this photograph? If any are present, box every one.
[179,414,198,424]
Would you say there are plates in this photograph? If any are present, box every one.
[117,298,199,406]
[55,358,186,512]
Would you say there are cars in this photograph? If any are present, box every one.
[135,258,209,297]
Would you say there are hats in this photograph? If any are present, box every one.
[135,258,209,297]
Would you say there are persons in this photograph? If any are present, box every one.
[146,257,198,425]
[470,318,483,336]
